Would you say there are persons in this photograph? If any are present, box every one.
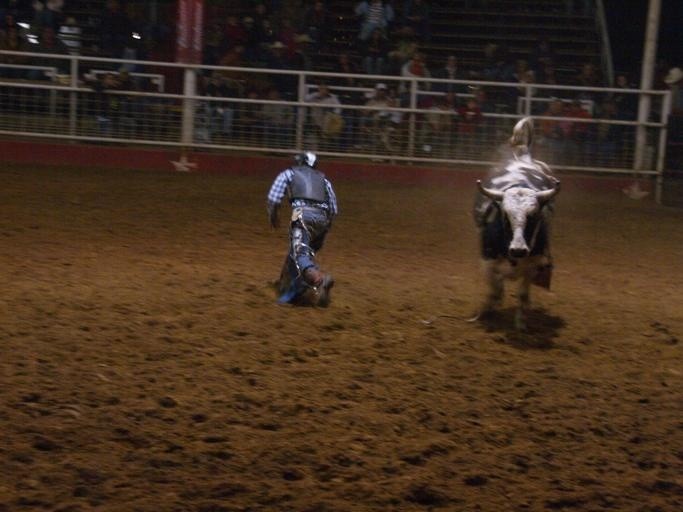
[1,0,682,167]
[264,151,339,309]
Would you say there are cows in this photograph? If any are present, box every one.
[473,116,557,331]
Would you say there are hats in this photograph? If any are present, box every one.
[664,68,683,83]
[270,42,285,49]
[374,83,388,91]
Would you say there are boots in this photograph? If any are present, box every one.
[304,268,334,308]
